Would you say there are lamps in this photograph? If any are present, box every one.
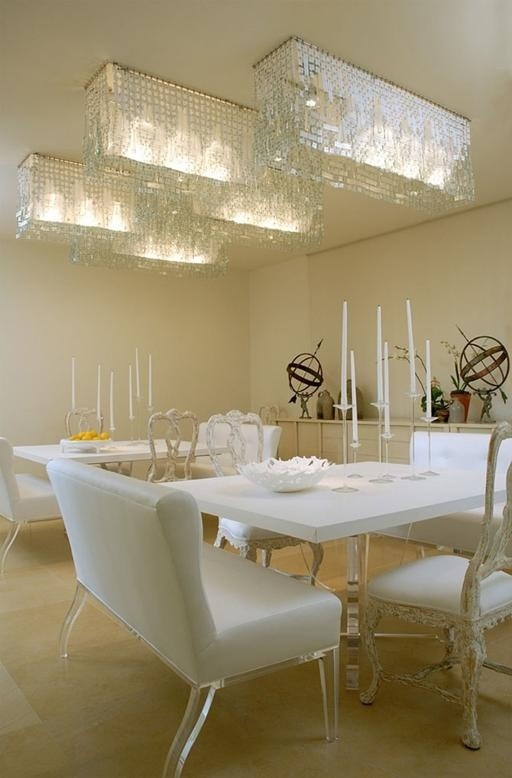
[250,37,474,220]
[14,151,232,280]
[83,61,326,252]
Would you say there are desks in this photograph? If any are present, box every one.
[12,439,229,478]
[156,462,511,692]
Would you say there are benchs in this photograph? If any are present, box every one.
[197,422,282,477]
[45,458,343,777]
[359,431,510,591]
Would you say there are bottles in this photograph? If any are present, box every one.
[447,397,466,424]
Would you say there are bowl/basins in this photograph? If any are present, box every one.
[60,438,113,450]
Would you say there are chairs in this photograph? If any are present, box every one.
[146,410,199,484]
[1,437,72,573]
[357,421,510,751]
[203,411,325,586]
[65,409,103,443]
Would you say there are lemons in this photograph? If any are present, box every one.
[70,431,109,440]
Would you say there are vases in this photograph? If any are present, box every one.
[434,392,471,423]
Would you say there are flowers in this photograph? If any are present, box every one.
[377,341,508,412]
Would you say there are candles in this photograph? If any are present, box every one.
[70,347,153,429]
[341,298,432,447]
